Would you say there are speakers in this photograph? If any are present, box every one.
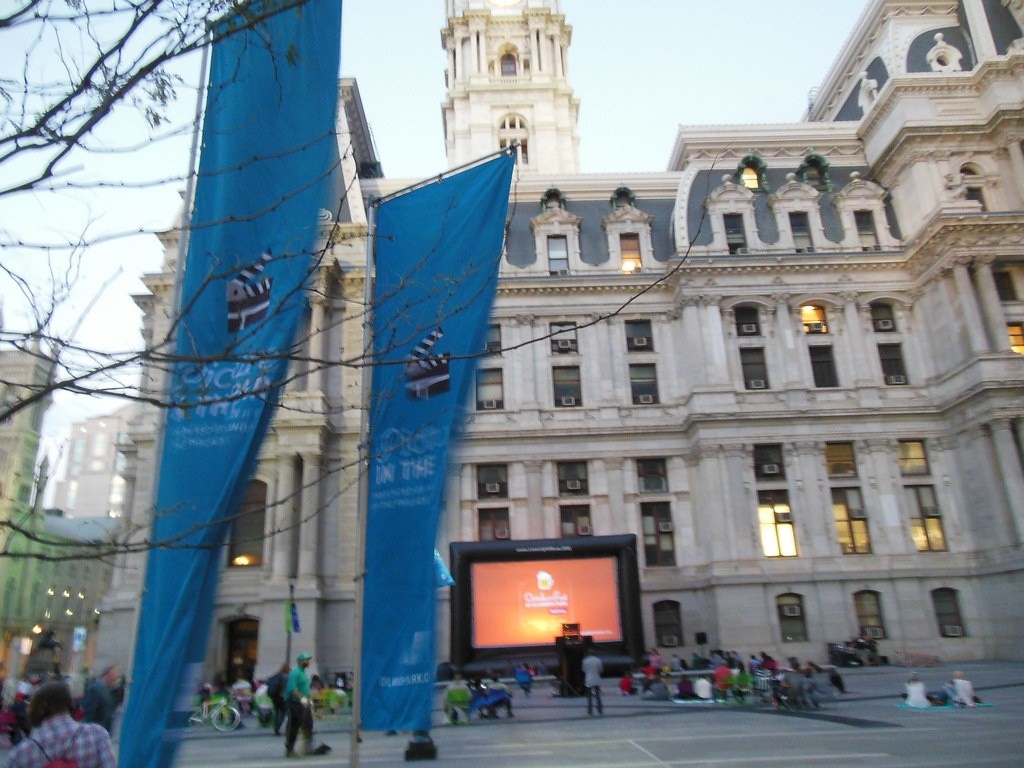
[695,632,708,645]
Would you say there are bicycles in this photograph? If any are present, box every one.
[186,686,241,733]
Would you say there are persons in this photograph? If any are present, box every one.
[801,667,814,692]
[514,662,533,697]
[0,681,117,768]
[201,671,356,729]
[857,633,883,666]
[266,663,290,735]
[0,662,125,751]
[485,671,514,718]
[465,675,500,720]
[770,656,818,711]
[580,647,606,715]
[445,666,472,726]
[641,647,778,702]
[284,653,316,759]
[619,672,637,695]
[904,669,931,709]
[807,659,862,702]
[941,670,977,707]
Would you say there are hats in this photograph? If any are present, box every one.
[296,652,313,662]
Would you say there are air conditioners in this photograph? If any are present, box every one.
[494,528,509,539]
[577,525,592,536]
[762,464,780,474]
[486,484,500,493]
[743,324,757,333]
[631,268,643,276]
[562,396,576,406]
[567,480,580,490]
[640,395,653,403]
[782,606,802,616]
[868,244,882,252]
[879,320,893,329]
[924,506,941,516]
[483,399,497,409]
[943,624,962,637]
[804,247,816,252]
[480,342,487,350]
[662,636,678,646]
[891,376,905,384]
[775,513,793,523]
[750,380,766,388]
[736,248,750,254]
[809,323,821,332]
[634,337,646,346]
[558,340,571,348]
[558,270,570,275]
[864,627,884,640]
[659,521,672,533]
[851,509,866,519]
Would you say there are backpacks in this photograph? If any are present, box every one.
[27,721,83,768]
